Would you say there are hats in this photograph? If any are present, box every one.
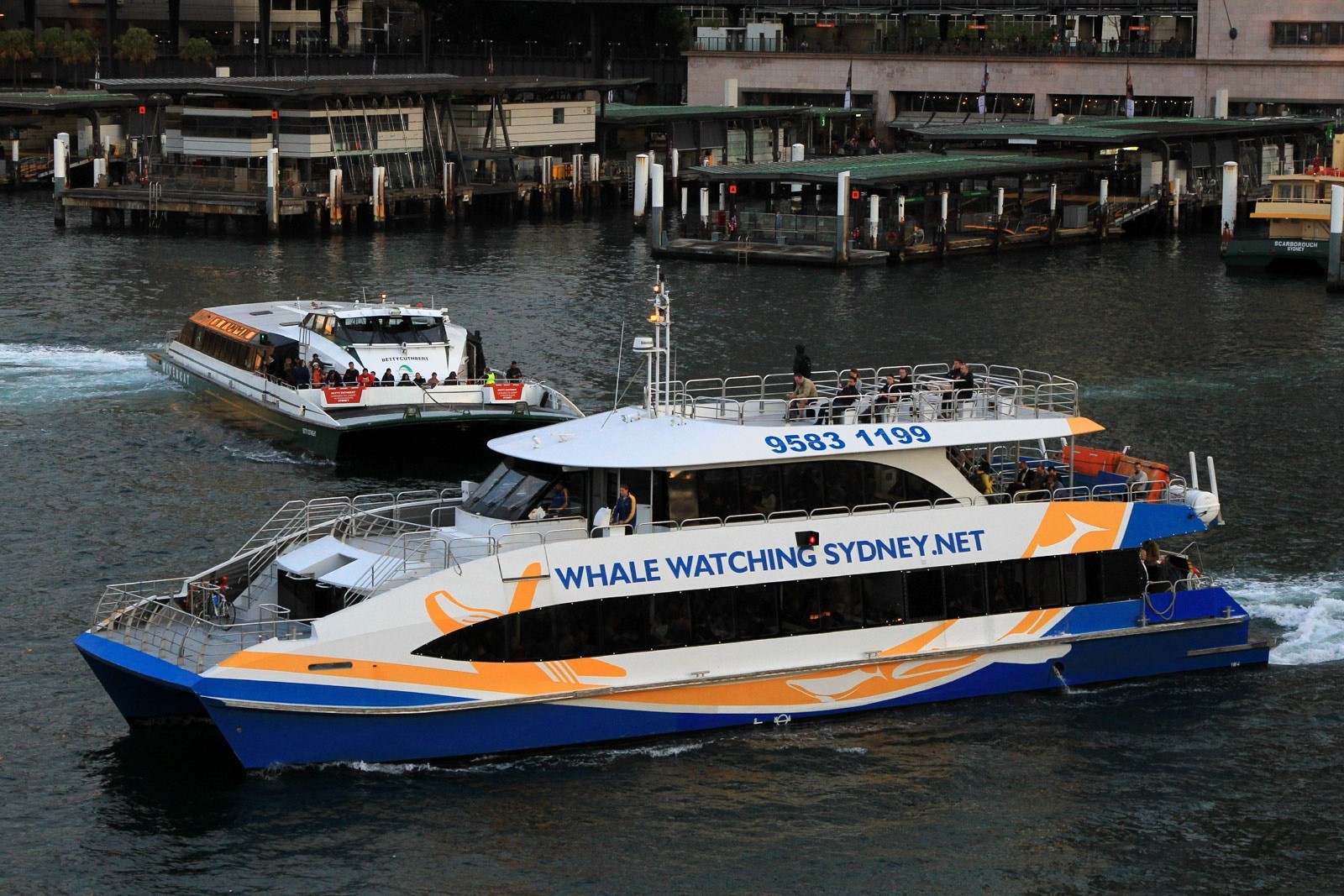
[312,362,319,366]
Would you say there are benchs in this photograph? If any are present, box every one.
[1060,472,1152,499]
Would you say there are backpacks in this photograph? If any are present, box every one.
[973,472,987,493]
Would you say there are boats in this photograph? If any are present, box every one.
[88,262,1289,775]
[1219,134,1344,288]
[145,282,589,462]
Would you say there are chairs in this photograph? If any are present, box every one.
[801,374,1017,424]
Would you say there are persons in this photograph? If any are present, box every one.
[548,480,572,516]
[264,353,496,390]
[946,446,1068,502]
[783,345,975,425]
[609,484,637,534]
[1139,539,1160,595]
[954,35,1185,57]
[850,225,925,250]
[1123,462,1148,502]
[505,361,523,383]
